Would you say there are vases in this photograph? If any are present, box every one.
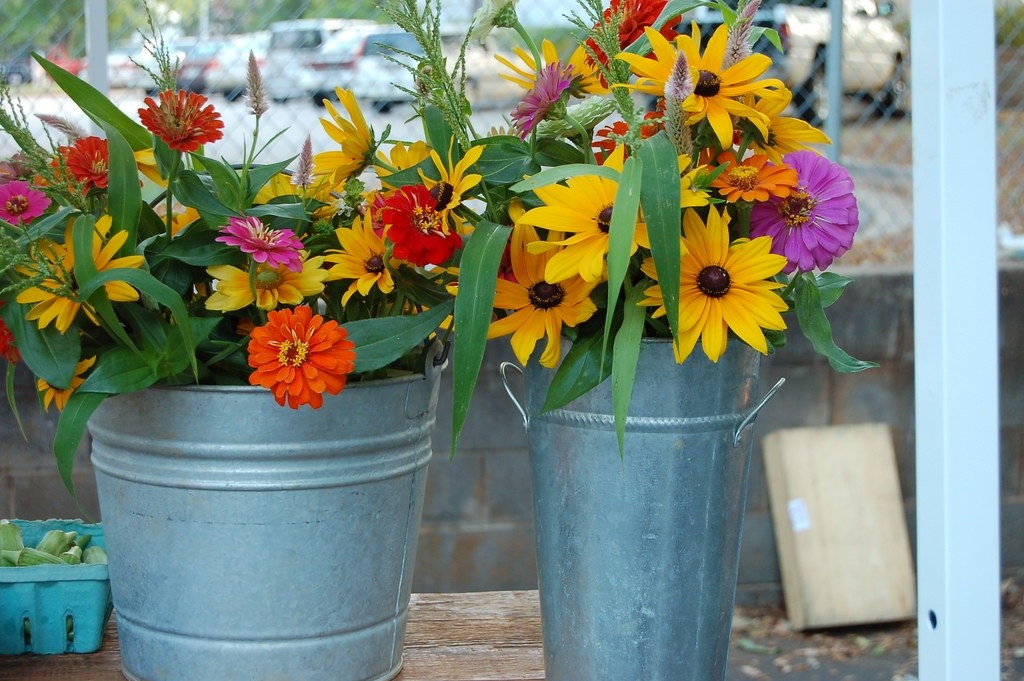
[498,336,789,681]
[85,335,452,680]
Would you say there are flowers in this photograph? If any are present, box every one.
[0,1,884,498]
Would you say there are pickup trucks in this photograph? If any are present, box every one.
[673,0,913,121]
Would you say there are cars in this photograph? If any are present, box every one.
[1,15,504,115]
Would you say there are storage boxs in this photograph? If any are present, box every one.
[760,424,916,631]
[0,517,112,657]
